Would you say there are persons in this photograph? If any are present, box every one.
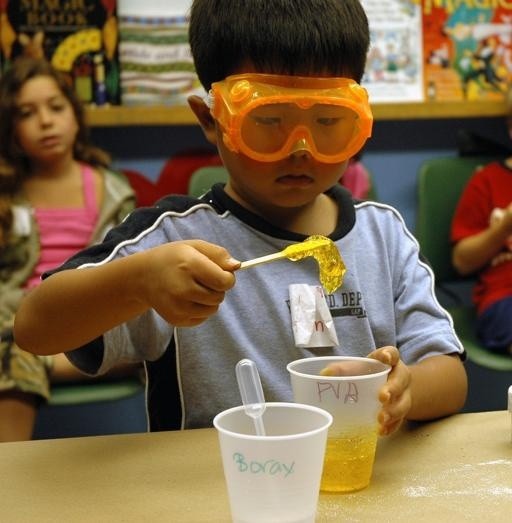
[0,59,139,445]
[1,156,50,444]
[451,123,512,359]
[13,0,469,437]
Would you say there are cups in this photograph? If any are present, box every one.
[211,399,334,523]
[284,353,394,494]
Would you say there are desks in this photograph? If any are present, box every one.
[1,409,512,523]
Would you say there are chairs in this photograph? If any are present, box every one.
[416,156,511,384]
[188,166,378,202]
[46,171,147,405]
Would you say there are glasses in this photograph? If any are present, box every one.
[204,73,374,167]
